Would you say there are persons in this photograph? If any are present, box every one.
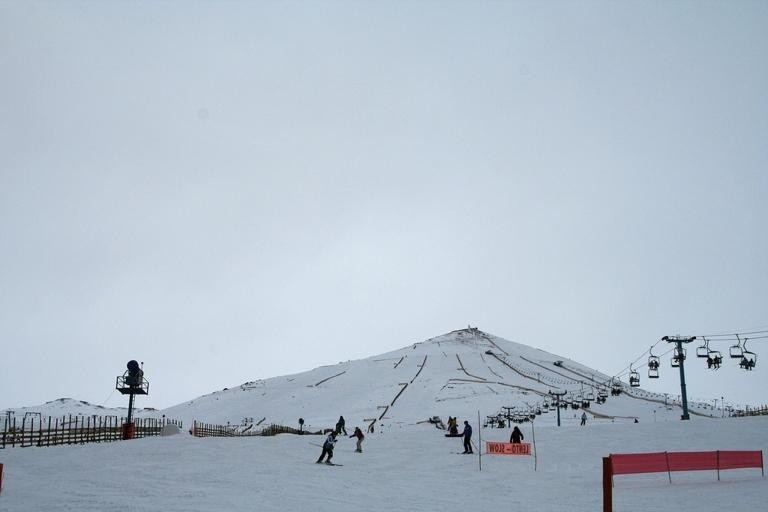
[675,353,684,362]
[634,418,638,424]
[504,401,558,424]
[510,426,523,443]
[349,427,365,452]
[446,416,459,437]
[581,412,587,426]
[316,430,338,464]
[707,355,719,369]
[459,421,473,454]
[339,415,347,435]
[561,388,621,410]
[742,357,754,370]
[650,360,659,369]
[630,376,634,384]
